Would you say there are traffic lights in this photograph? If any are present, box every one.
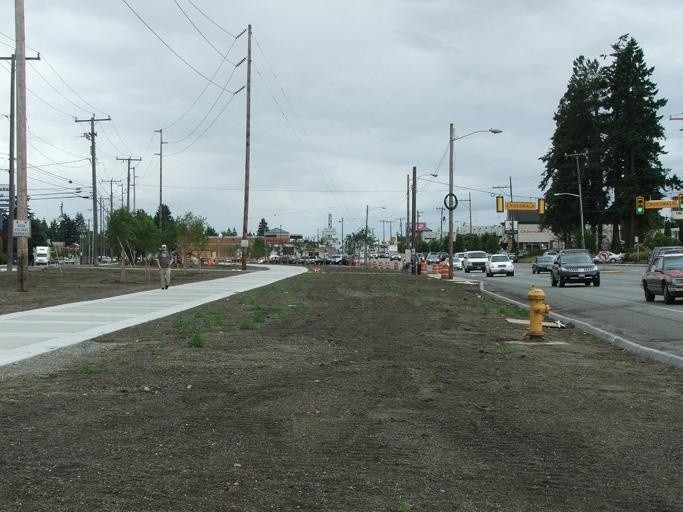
[636,196,644,214]
[679,193,683,209]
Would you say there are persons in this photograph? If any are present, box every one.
[157,244,173,289]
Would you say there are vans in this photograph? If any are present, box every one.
[462,251,487,273]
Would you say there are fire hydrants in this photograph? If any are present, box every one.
[526,285,552,336]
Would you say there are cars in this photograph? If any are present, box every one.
[645,246,683,267]
[97,255,117,263]
[128,249,402,266]
[638,253,683,305]
[530,256,553,274]
[486,254,515,278]
[548,251,600,288]
[414,249,622,270]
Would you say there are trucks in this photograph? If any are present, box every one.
[32,245,52,266]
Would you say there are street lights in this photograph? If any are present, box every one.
[406,172,438,263]
[448,121,503,279]
[364,205,388,264]
[552,192,585,248]
